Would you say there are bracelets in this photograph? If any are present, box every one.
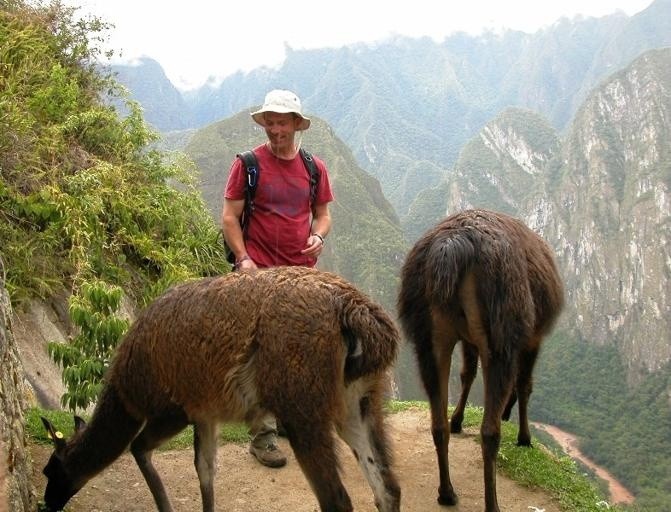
[235,254,251,267]
[312,232,324,245]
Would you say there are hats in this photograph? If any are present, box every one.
[252,89,311,132]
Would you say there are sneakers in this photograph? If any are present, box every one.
[250,433,286,467]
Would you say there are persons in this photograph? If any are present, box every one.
[221,90,335,468]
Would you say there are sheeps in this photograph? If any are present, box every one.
[39,264,403,512]
[394,205,567,512]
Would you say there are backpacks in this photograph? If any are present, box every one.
[226,148,319,261]
